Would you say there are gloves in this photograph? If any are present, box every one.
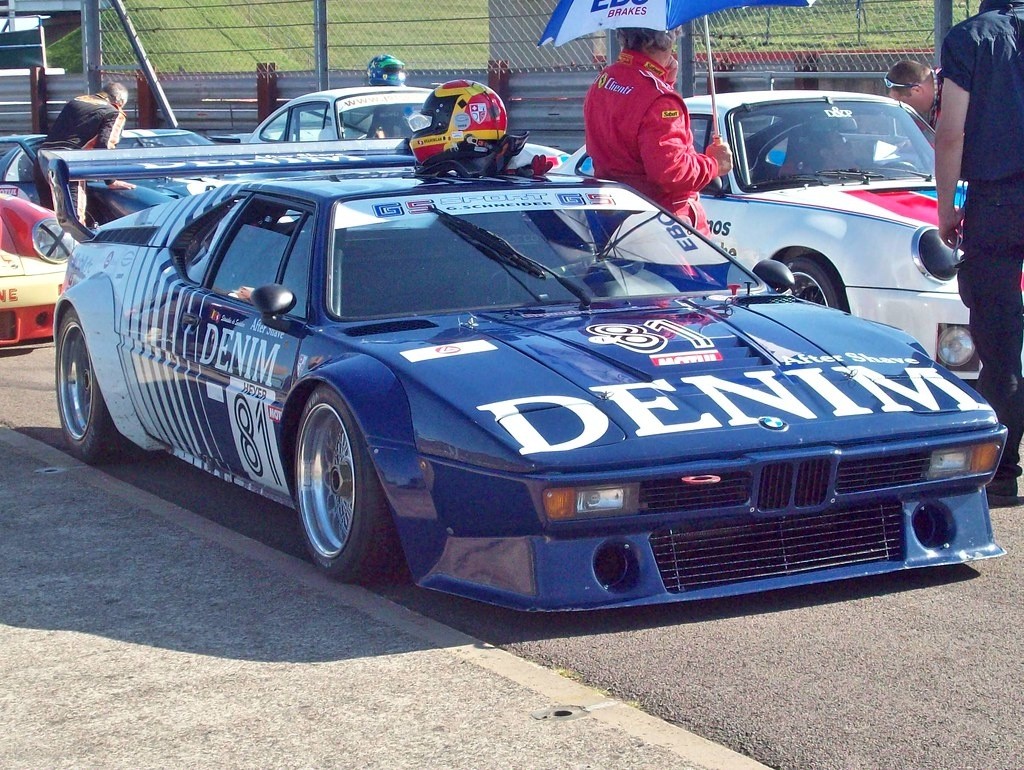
[505,155,553,179]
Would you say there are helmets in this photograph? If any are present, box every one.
[808,112,857,149]
[409,79,508,168]
[366,55,406,86]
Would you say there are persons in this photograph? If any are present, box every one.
[882,60,942,128]
[584,27,733,284]
[812,119,858,173]
[36,80,136,226]
[934,0,1024,500]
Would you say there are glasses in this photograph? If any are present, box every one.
[884,72,919,89]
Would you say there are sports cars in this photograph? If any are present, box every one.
[523,89,1024,388]
[0,84,591,347]
[37,138,1008,612]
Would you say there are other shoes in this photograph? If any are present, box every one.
[985,474,1018,507]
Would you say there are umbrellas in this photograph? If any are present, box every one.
[536,0,815,145]
